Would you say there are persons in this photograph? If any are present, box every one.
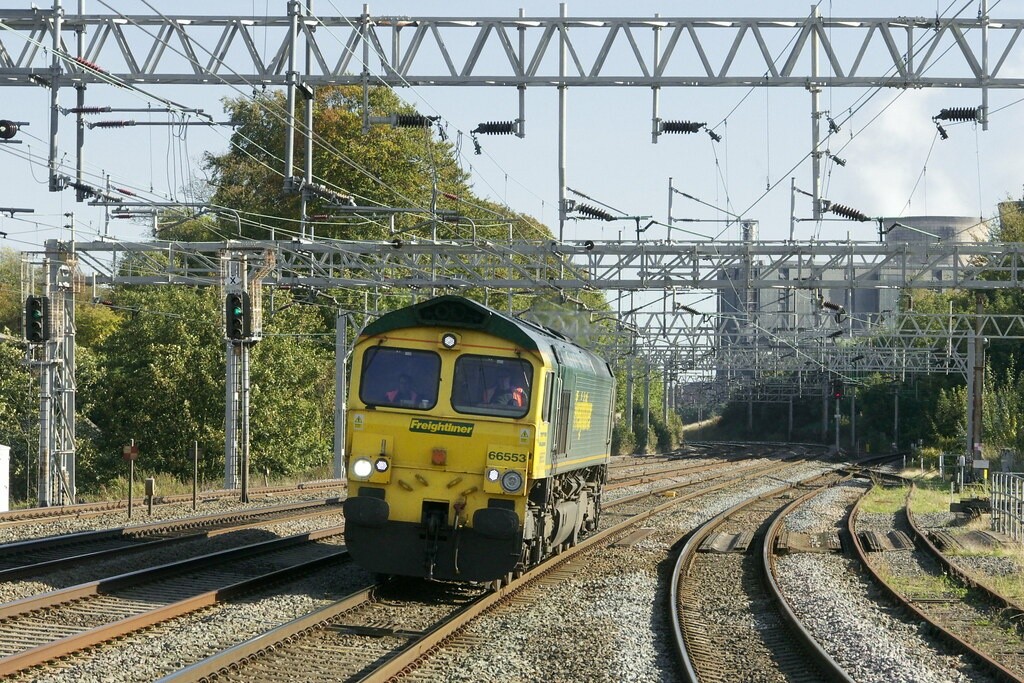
[484,368,528,407]
[384,375,421,404]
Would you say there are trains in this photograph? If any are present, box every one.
[343,294,616,592]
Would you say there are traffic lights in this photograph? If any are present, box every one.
[226,292,244,341]
[833,379,845,399]
[25,295,49,342]
